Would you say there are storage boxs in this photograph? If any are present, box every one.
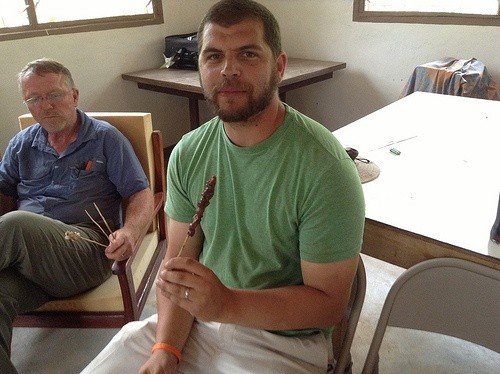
[164,32,199,71]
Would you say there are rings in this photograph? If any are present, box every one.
[121,254,124,258]
[184,287,190,300]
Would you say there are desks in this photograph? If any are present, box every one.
[332,92,500,361]
[121,57,347,131]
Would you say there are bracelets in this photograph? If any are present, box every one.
[151,342,182,365]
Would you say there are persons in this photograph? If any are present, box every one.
[79,0,365,374]
[0,57,155,374]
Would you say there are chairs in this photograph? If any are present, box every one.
[325,255,500,374]
[10,113,166,329]
